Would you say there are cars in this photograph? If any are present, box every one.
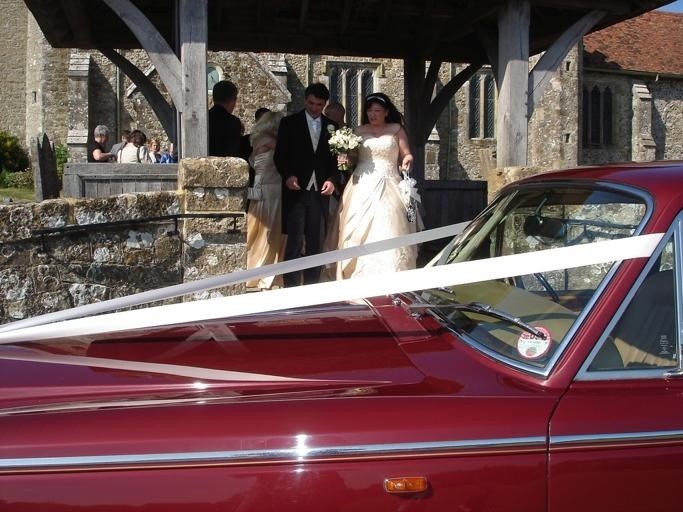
[0,160,683,512]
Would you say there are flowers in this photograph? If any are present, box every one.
[327,123,336,135]
[328,126,363,171]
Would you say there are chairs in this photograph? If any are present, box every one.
[614,270,678,369]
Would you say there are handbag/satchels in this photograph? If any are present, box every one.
[397,169,421,222]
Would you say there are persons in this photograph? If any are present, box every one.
[87,125,117,162]
[147,137,161,163]
[323,101,347,129]
[274,82,342,288]
[245,110,286,291]
[159,139,177,164]
[207,79,242,157]
[114,130,157,164]
[108,131,129,162]
[318,91,427,284]
[242,106,271,158]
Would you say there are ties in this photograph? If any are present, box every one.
[311,120,318,151]
